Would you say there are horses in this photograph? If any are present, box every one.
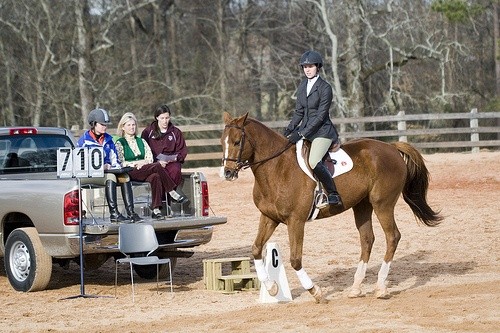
[218,110,451,303]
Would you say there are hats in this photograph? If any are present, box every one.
[87,108,112,126]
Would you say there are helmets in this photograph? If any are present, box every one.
[298,51,324,68]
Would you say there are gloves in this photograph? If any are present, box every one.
[288,131,302,144]
[283,128,291,136]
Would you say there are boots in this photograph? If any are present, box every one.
[121,181,145,223]
[314,161,340,204]
[105,179,134,222]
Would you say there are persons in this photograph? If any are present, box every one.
[114,112,186,219]
[141,105,187,216]
[283,51,342,206]
[75,109,146,224]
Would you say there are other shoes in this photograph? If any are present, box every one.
[171,194,186,203]
[151,212,162,218]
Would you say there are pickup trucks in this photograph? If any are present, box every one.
[0,126,228,292]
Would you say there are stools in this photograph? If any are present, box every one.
[201,256,251,291]
[216,274,260,291]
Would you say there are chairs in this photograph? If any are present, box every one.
[130,182,152,217]
[19,150,39,168]
[162,192,184,218]
[2,152,19,167]
[114,224,173,304]
[81,183,109,221]
